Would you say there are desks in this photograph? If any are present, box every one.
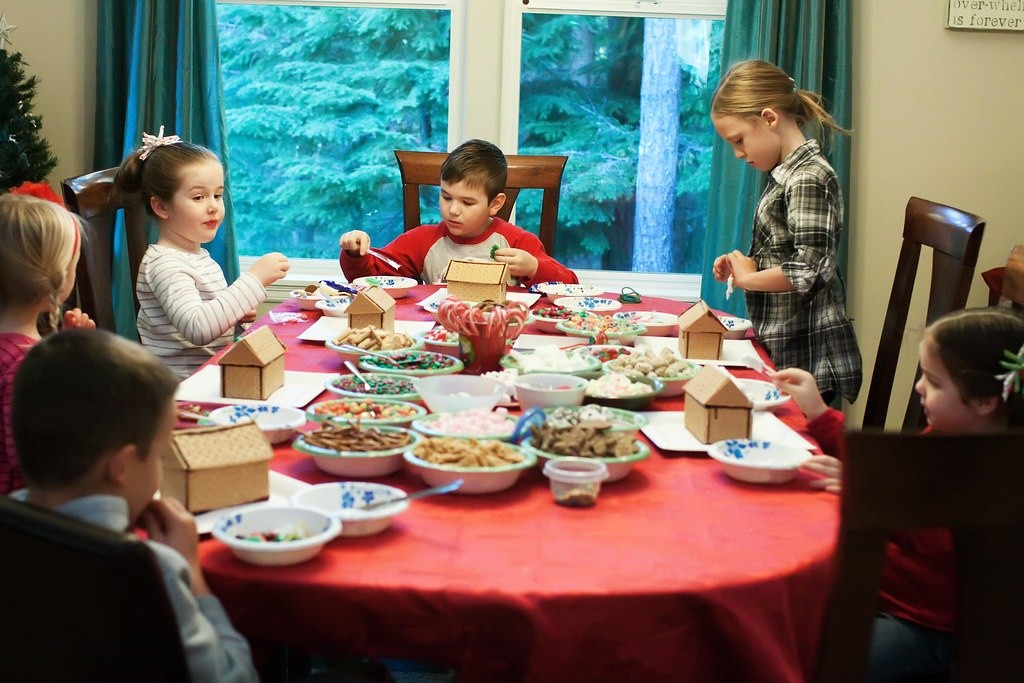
[161,284,849,683]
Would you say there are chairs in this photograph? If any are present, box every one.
[0,495,193,683]
[60,163,152,340]
[861,199,988,432]
[819,427,1024,683]
[394,149,568,283]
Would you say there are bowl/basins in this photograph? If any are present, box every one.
[707,437,814,483]
[718,315,752,339]
[736,377,792,413]
[288,481,411,536]
[287,277,700,508]
[210,405,307,443]
[211,504,343,565]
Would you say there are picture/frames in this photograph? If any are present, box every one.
[945,0,1024,32]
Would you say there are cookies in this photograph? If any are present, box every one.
[343,286,396,331]
[160,419,273,512]
[217,324,287,399]
[681,364,753,444]
[472,299,522,313]
[333,325,412,351]
[291,420,639,466]
[444,258,510,302]
[305,285,318,292]
[678,299,727,359]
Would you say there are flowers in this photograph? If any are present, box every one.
[995,343,1024,401]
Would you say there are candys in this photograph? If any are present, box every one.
[244,532,301,541]
[534,305,639,362]
[489,244,500,259]
[423,325,460,342]
[314,353,453,419]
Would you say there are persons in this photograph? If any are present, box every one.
[340,140,579,288]
[0,194,257,683]
[105,125,290,382]
[711,59,863,406]
[770,307,1024,683]
